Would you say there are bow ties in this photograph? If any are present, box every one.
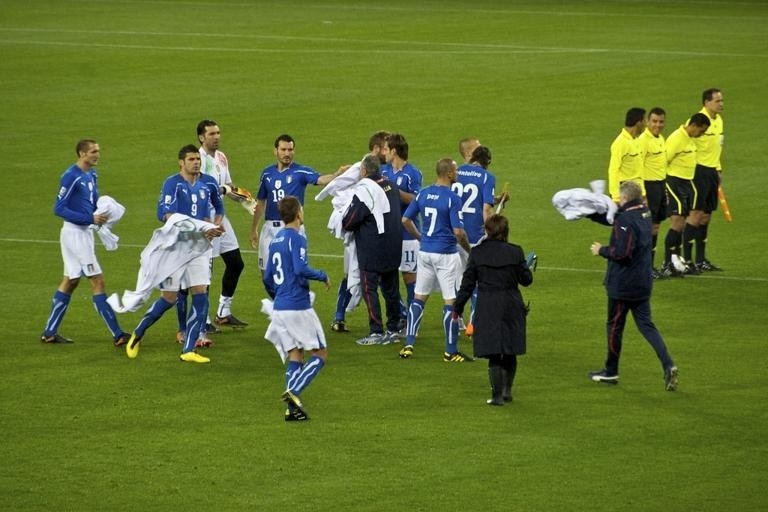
[194,338,214,348]
[204,324,224,335]
[40,334,74,344]
[126,331,140,359]
[175,332,188,344]
[113,333,132,347]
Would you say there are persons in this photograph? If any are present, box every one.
[263,196,331,420]
[585,181,680,392]
[664,113,711,278]
[331,132,392,332]
[684,88,725,276]
[636,107,667,279]
[249,134,351,320]
[452,213,533,406]
[453,138,510,331]
[125,145,224,364]
[608,108,647,215]
[195,120,257,335]
[176,280,213,349]
[41,140,132,348]
[401,159,476,364]
[385,134,424,338]
[339,154,404,346]
[452,147,495,336]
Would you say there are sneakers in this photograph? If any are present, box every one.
[213,314,248,328]
[284,407,292,419]
[398,329,408,338]
[280,390,309,421]
[695,262,723,272]
[486,398,504,405]
[678,261,701,275]
[356,333,382,346]
[661,264,680,276]
[330,322,352,332]
[665,367,679,392]
[458,327,468,336]
[443,352,474,363]
[590,370,619,385]
[650,268,665,279]
[671,262,690,274]
[399,346,414,358]
[180,350,210,363]
[381,331,400,345]
[502,394,513,402]
